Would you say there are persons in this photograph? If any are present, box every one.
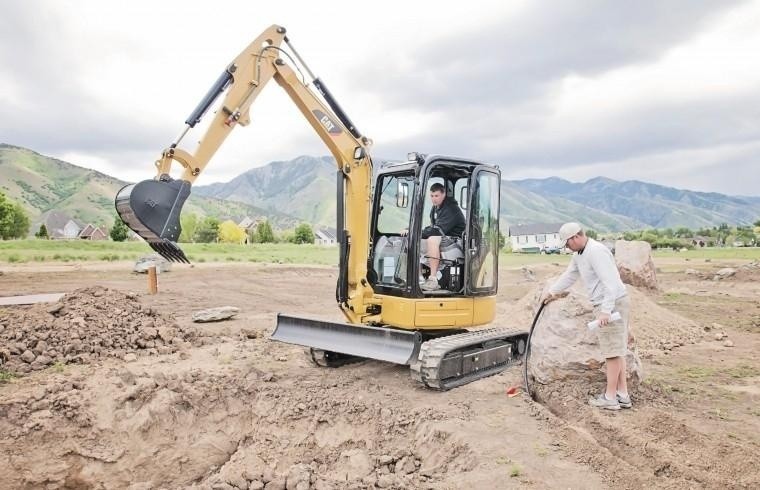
[398,183,467,293]
[539,221,633,411]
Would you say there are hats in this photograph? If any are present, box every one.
[557,222,582,249]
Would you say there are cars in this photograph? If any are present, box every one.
[540,245,560,255]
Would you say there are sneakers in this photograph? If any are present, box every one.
[588,393,620,411]
[616,392,633,408]
[421,277,439,291]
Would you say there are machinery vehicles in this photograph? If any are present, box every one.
[113,24,532,393]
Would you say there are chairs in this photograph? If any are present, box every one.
[421,236,465,271]
